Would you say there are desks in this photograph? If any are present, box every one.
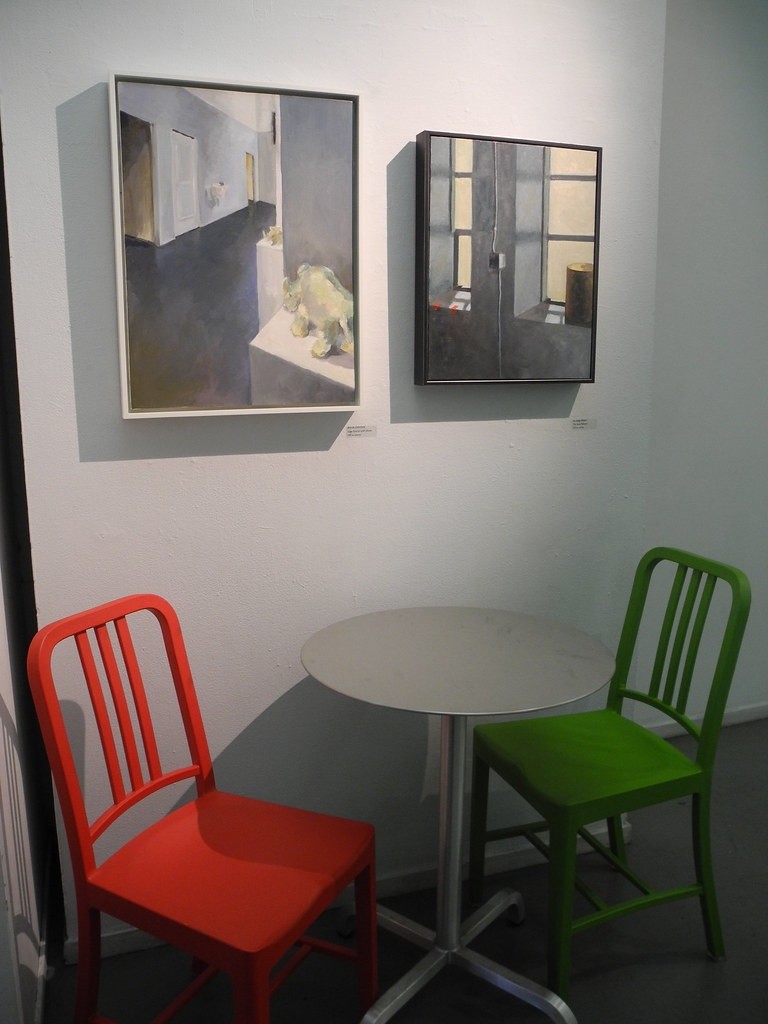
[301,605,617,1024]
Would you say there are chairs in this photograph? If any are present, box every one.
[472,544,753,996]
[24,593,380,1024]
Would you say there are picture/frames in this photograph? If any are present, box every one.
[110,72,363,417]
[414,130,603,386]
